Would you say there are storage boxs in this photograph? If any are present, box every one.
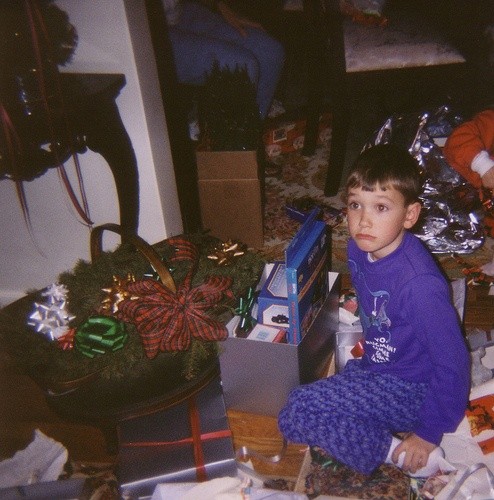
[195,136,269,250]
[220,267,342,418]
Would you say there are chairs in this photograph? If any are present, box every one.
[299,1,473,196]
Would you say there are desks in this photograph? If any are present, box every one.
[43,343,232,483]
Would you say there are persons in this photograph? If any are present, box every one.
[160,0,291,122]
[278,144,474,475]
[442,109,494,193]
